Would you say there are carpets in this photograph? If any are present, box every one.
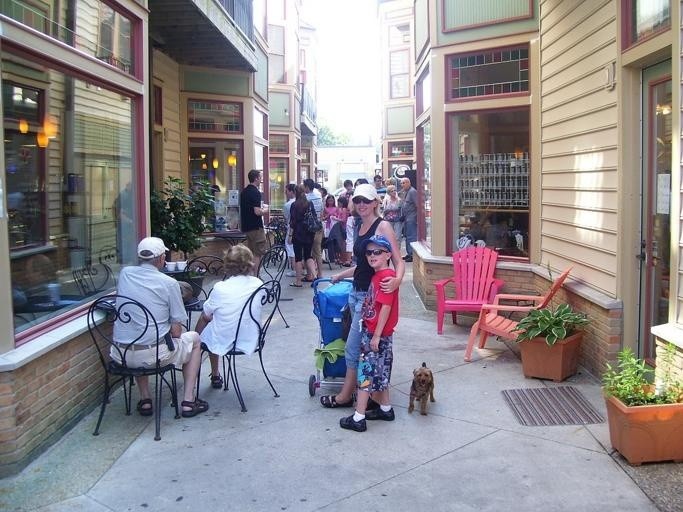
[500,386,606,427]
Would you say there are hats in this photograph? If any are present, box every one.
[351,184,377,201]
[362,234,391,251]
[137,236,170,260]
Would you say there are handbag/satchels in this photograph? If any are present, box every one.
[383,210,402,222]
[303,209,323,233]
[178,280,194,304]
[340,303,352,341]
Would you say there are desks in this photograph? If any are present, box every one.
[15,294,87,324]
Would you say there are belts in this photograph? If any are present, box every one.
[116,340,164,350]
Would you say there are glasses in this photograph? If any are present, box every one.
[353,197,374,203]
[365,249,388,255]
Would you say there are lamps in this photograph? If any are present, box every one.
[18,110,59,149]
[199,153,236,170]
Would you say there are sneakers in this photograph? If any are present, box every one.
[301,271,307,275]
[365,407,395,421]
[287,271,296,276]
[322,256,352,267]
[403,255,413,262]
[340,414,366,432]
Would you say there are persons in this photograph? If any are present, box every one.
[340,235,400,432]
[112,183,134,263]
[194,244,266,390]
[25,254,69,298]
[320,183,406,410]
[109,236,208,417]
[240,170,417,288]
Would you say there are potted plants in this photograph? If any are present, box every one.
[599,344,683,467]
[509,302,593,384]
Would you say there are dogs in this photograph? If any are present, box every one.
[408,362,436,415]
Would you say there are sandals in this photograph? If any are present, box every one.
[320,395,354,407]
[367,397,380,410]
[211,373,223,389]
[181,397,209,417]
[137,399,152,416]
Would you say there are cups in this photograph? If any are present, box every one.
[47,283,61,304]
[165,261,176,271]
[176,260,188,271]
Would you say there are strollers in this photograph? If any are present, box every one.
[309,279,356,402]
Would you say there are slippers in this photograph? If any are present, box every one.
[301,277,313,282]
[289,282,302,287]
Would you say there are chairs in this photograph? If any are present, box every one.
[463,265,573,363]
[433,244,505,337]
